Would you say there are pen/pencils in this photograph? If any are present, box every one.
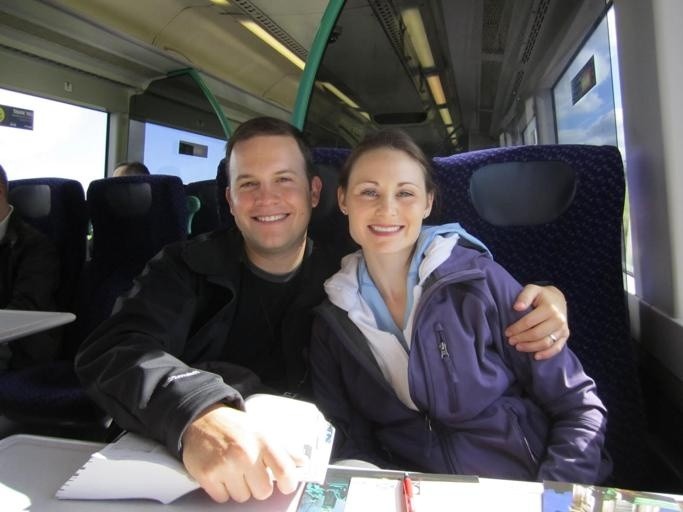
[403,473,415,512]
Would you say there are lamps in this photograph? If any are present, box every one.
[213,0,471,146]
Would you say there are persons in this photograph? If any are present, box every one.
[71,114,572,503]
[307,130,613,486]
[112,161,150,178]
[0,163,62,438]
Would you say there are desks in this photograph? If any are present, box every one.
[0,431,683,512]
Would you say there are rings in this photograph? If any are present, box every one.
[550,334,558,343]
